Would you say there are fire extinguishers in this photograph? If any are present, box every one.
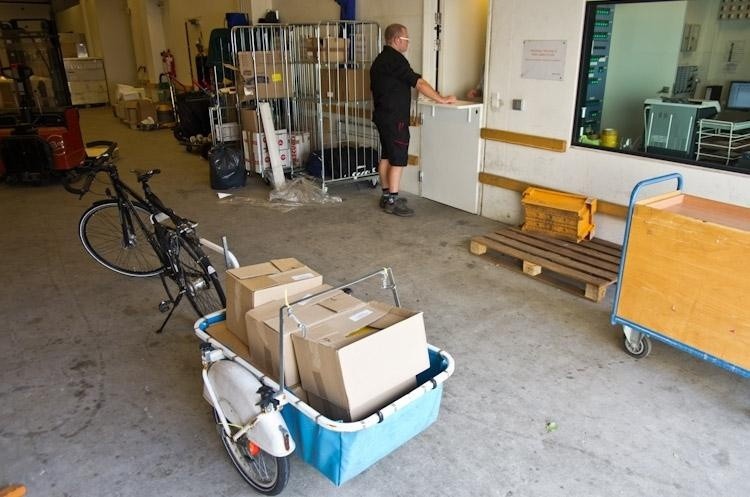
[161,49,177,78]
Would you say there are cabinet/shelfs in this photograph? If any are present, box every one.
[695,114,750,171]
[64,54,115,112]
[208,17,385,195]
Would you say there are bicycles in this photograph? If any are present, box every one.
[57,137,228,335]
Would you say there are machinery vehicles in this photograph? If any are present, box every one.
[0,16,119,186]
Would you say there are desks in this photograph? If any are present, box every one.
[638,95,721,159]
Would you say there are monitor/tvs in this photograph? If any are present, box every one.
[726,81,750,111]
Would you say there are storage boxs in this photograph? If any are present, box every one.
[612,186,750,377]
[215,34,374,178]
[206,254,434,422]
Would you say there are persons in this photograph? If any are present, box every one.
[368,22,460,217]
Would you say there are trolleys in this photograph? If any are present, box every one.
[157,69,211,154]
[228,20,303,183]
[283,17,384,196]
[606,173,749,384]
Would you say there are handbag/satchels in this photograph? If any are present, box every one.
[309,147,378,178]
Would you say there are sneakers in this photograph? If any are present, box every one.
[380,196,414,217]
